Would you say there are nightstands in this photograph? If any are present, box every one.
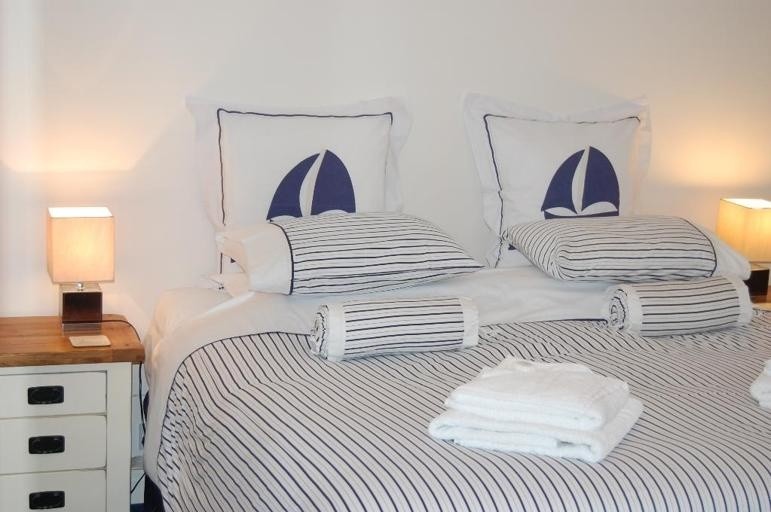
[0,314,145,512]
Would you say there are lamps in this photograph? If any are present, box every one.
[715,199,771,295]
[46,205,116,335]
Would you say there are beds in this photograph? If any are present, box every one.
[146,288,771,512]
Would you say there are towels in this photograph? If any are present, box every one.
[427,398,644,465]
[444,354,631,432]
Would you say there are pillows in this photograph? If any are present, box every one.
[310,295,479,362]
[603,275,754,338]
[463,90,648,270]
[214,210,486,295]
[504,216,729,281]
[209,96,404,292]
